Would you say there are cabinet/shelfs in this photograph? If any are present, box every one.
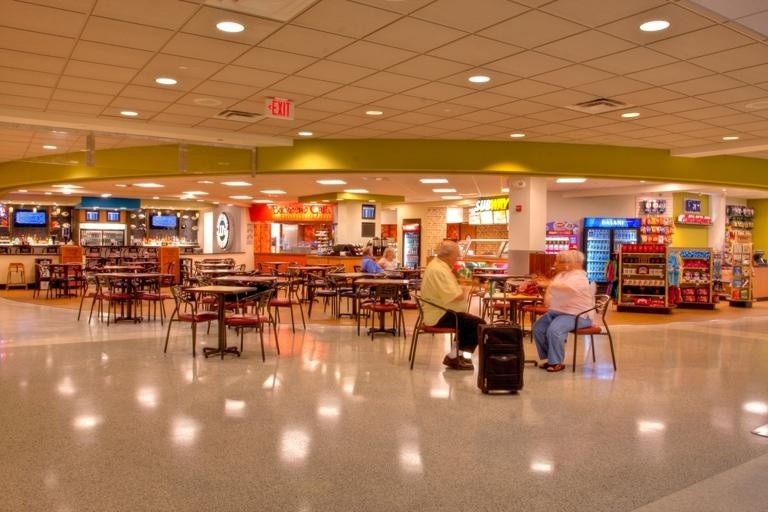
[670,250,715,307]
[619,243,669,312]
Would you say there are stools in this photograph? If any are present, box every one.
[6,262,28,291]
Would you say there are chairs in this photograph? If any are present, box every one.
[565,294,617,371]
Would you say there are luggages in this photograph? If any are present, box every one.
[477,278,524,394]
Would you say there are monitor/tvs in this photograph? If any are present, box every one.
[149,213,180,230]
[107,211,120,222]
[85,211,99,221]
[14,209,47,227]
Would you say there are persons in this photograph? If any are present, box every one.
[361,246,386,274]
[533,249,595,372]
[378,247,411,302]
[418,239,487,370]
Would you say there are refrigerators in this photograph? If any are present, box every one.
[584,217,640,290]
[401,223,420,290]
[78,223,126,274]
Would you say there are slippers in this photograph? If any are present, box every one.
[539,363,565,372]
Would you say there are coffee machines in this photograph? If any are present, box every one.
[381,237,389,255]
[372,237,381,256]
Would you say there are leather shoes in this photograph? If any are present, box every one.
[443,356,474,370]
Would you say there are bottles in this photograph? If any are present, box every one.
[545,239,568,250]
[406,232,418,268]
[616,230,638,251]
[586,230,609,284]
[142,234,186,246]
[621,244,665,253]
[11,232,50,244]
